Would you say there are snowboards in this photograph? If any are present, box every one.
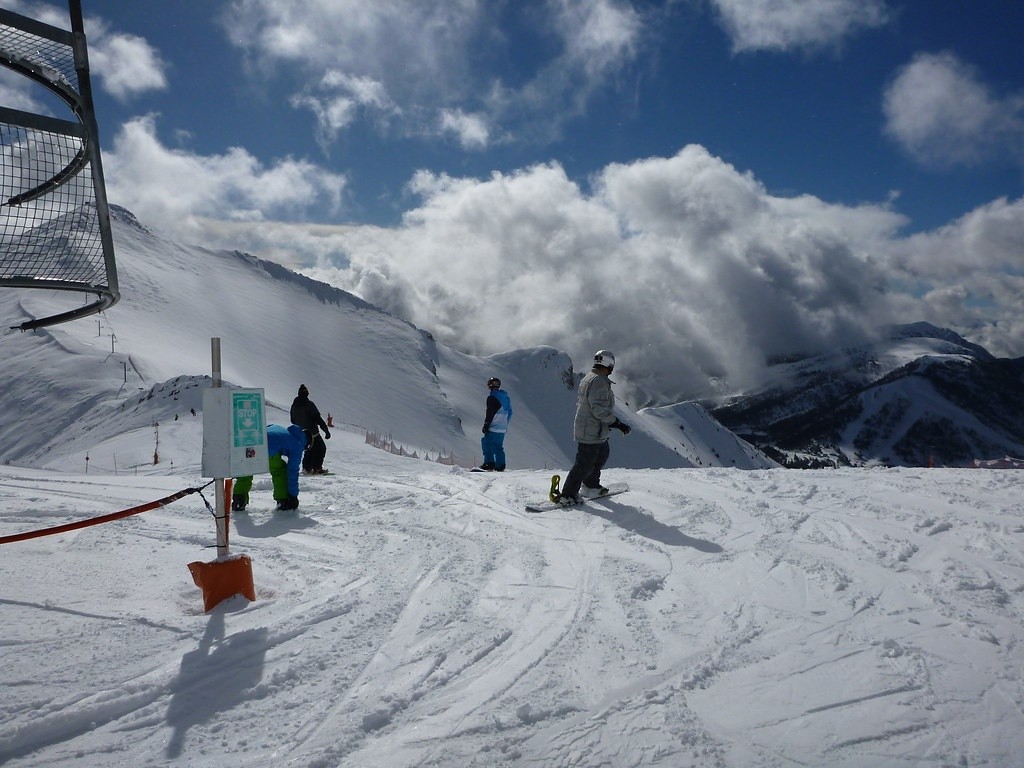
[523,481,629,512]
[468,467,498,472]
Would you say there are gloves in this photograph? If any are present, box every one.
[325,431,330,439]
[608,417,632,437]
[287,493,297,511]
[482,423,489,433]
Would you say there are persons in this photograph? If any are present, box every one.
[231,424,314,511]
[479,378,512,471]
[191,407,196,416]
[562,349,631,503]
[327,413,333,426]
[174,414,178,421]
[290,384,331,474]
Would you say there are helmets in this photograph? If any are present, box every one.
[593,349,616,368]
[487,377,501,389]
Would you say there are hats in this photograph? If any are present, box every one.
[302,429,314,446]
[298,384,309,396]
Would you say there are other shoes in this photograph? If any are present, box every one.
[232,493,245,511]
[277,501,288,511]
[581,482,609,498]
[314,469,328,474]
[479,463,494,471]
[560,493,584,506]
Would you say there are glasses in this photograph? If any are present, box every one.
[303,444,312,452]
